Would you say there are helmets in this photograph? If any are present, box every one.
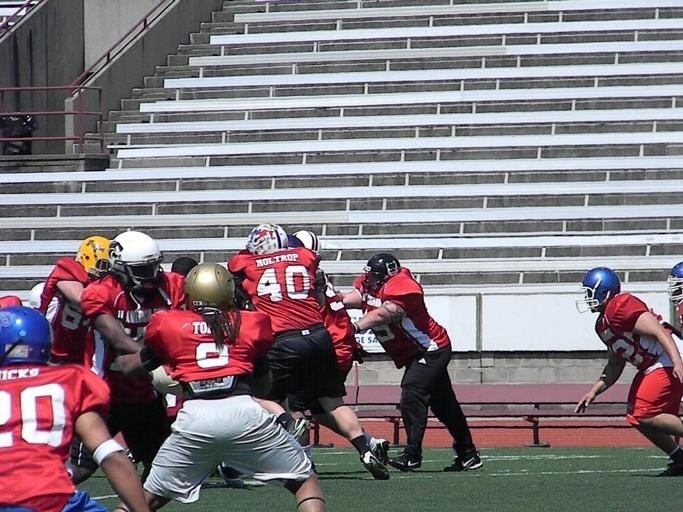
[576,267,620,313]
[75,236,112,278]
[185,264,235,312]
[287,234,305,249]
[294,229,321,258]
[246,222,288,254]
[362,254,400,292]
[172,257,196,275]
[29,283,58,310]
[0,306,53,363]
[669,262,683,306]
[109,231,164,292]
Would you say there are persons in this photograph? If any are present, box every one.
[227,223,389,488]
[0,306,148,512]
[113,261,326,512]
[39,236,109,368]
[293,229,322,256]
[288,235,391,468]
[341,253,484,473]
[574,267,683,477]
[666,263,683,338]
[64,230,185,488]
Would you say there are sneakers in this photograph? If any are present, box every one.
[656,462,680,477]
[217,462,245,488]
[370,437,389,467]
[289,419,310,446]
[443,450,483,472]
[360,451,390,480]
[386,454,421,469]
